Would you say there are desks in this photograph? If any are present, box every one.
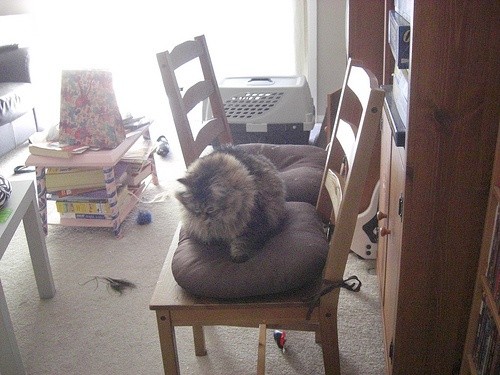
[0,180,56,375]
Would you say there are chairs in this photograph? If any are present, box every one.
[148,56,384,375]
[156,35,327,202]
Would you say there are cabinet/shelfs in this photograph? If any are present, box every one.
[460,136,500,375]
[346,0,500,375]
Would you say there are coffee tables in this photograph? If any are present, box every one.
[25,116,159,236]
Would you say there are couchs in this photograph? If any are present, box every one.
[0,43,44,157]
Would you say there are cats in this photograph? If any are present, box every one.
[175,145,286,263]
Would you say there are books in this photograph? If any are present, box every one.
[25,112,153,218]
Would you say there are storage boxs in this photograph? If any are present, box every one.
[44,160,153,218]
[380,0,413,147]
